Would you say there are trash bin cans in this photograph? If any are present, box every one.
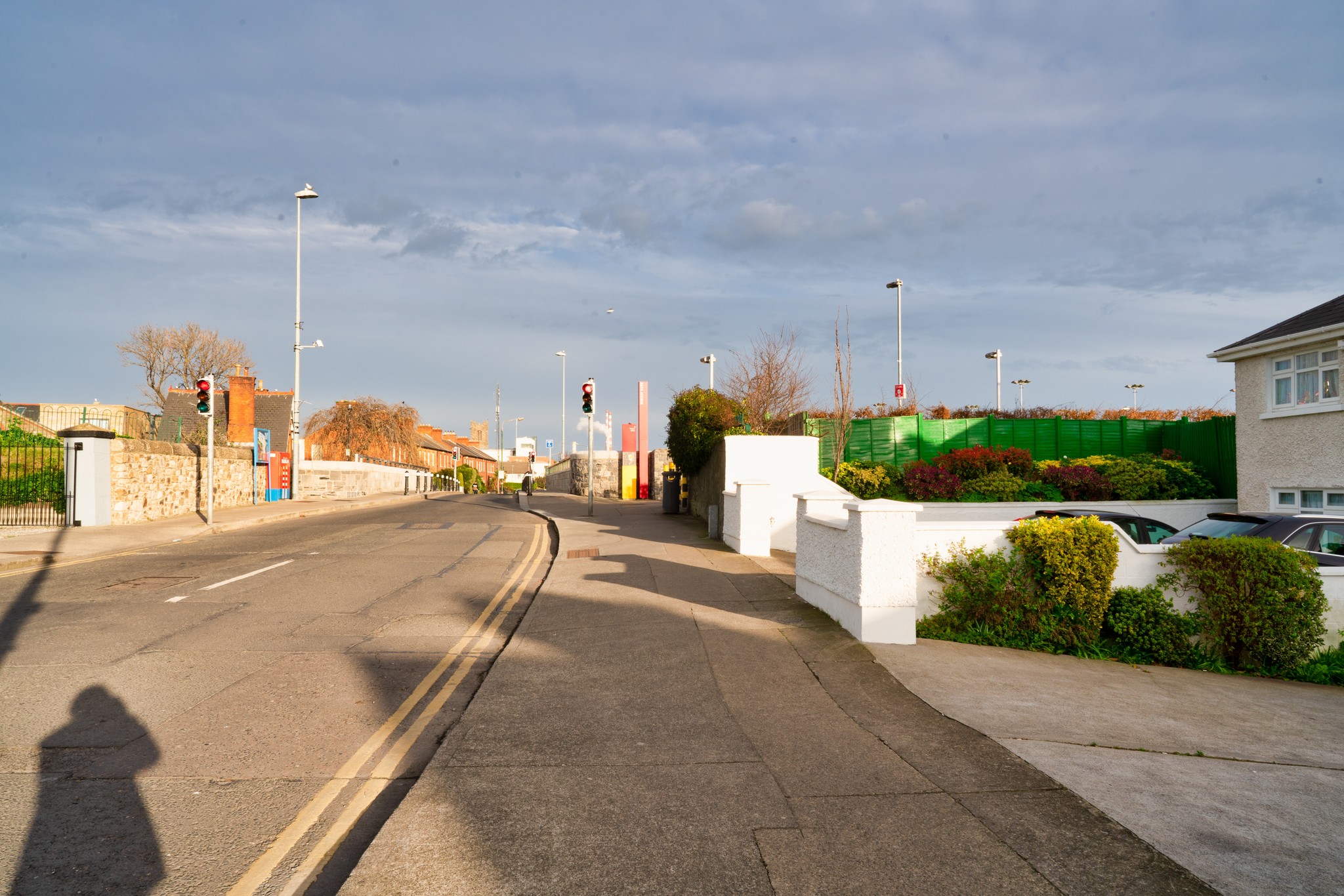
[662,471,680,514]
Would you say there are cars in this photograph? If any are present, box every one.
[536,489,545,491]
[515,489,523,494]
[1014,510,1179,547]
[1154,509,1344,565]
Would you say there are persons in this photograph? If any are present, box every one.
[472,481,479,494]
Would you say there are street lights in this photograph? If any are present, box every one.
[291,182,323,499]
[500,417,524,494]
[700,354,716,391]
[984,349,1002,414]
[555,350,565,460]
[1011,379,1031,417]
[886,279,905,410]
[1125,384,1145,412]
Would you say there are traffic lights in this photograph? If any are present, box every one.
[532,452,535,463]
[196,378,213,417]
[452,448,457,460]
[581,382,595,415]
[528,452,531,463]
